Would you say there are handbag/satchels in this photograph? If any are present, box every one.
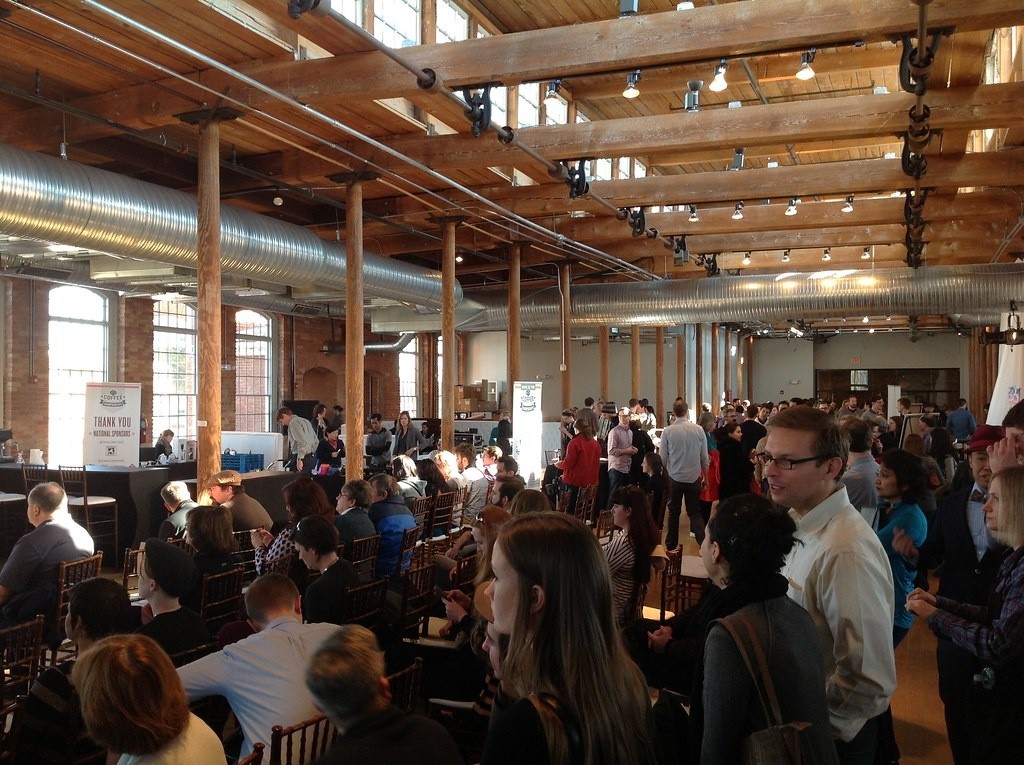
[705,613,815,765]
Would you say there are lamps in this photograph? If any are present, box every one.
[688,204,699,222]
[709,59,728,92]
[622,70,641,98]
[544,81,559,105]
[795,49,816,80]
[861,247,870,259]
[822,246,831,261]
[273,187,286,207]
[731,200,744,219]
[782,248,791,262]
[743,252,752,265]
[784,197,798,215]
[841,194,854,212]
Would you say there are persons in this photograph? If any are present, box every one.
[892,398,1024,765]
[599,484,663,628]
[0,481,95,701]
[470,509,688,765]
[275,407,320,473]
[70,634,228,765]
[0,578,130,764]
[364,411,442,468]
[310,404,346,471]
[175,573,346,765]
[513,392,980,516]
[876,448,928,652]
[368,444,527,592]
[306,624,464,765]
[154,429,174,462]
[754,406,900,765]
[438,505,512,642]
[130,468,275,669]
[496,420,512,458]
[698,493,839,765]
[251,475,398,624]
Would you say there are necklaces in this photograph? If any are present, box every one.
[321,556,339,574]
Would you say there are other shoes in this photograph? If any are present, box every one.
[439,619,454,637]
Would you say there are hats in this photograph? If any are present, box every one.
[145,537,199,598]
[473,581,494,624]
[204,470,242,487]
[962,424,1007,455]
[601,401,617,414]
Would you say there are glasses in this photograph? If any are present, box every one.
[756,452,834,470]
[339,493,350,499]
[475,512,485,524]
[877,401,884,404]
[296,522,314,544]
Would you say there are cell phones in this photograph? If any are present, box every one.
[434,585,451,601]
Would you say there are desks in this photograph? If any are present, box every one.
[0,458,303,570]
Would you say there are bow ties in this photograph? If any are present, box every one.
[970,488,989,504]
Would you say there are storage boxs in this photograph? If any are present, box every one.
[453,379,508,420]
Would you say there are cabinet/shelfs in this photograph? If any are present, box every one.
[475,380,502,409]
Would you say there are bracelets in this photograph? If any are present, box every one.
[297,458,303,461]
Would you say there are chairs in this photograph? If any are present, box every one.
[544,449,560,468]
[0,482,715,765]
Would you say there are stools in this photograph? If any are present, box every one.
[0,463,26,566]
[58,465,119,573]
[20,462,76,535]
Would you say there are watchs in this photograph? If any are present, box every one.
[413,446,417,451]
